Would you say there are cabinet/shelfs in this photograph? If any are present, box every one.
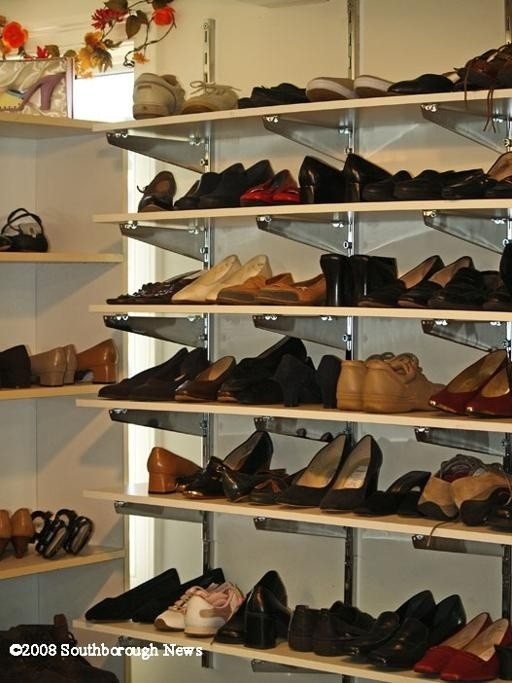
[75,86,511,680]
[2,113,136,680]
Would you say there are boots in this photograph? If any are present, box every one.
[0,614,119,683]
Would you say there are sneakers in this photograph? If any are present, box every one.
[132,43,512,131]
[185,588,246,638]
[152,583,218,633]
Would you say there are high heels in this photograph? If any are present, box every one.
[83,567,182,622]
[2,508,95,558]
[98,153,511,532]
[0,71,66,114]
[1,339,119,388]
[132,553,225,623]
[214,571,511,683]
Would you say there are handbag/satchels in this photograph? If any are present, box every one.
[2,207,49,253]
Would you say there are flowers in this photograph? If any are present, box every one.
[1,0,183,80]
